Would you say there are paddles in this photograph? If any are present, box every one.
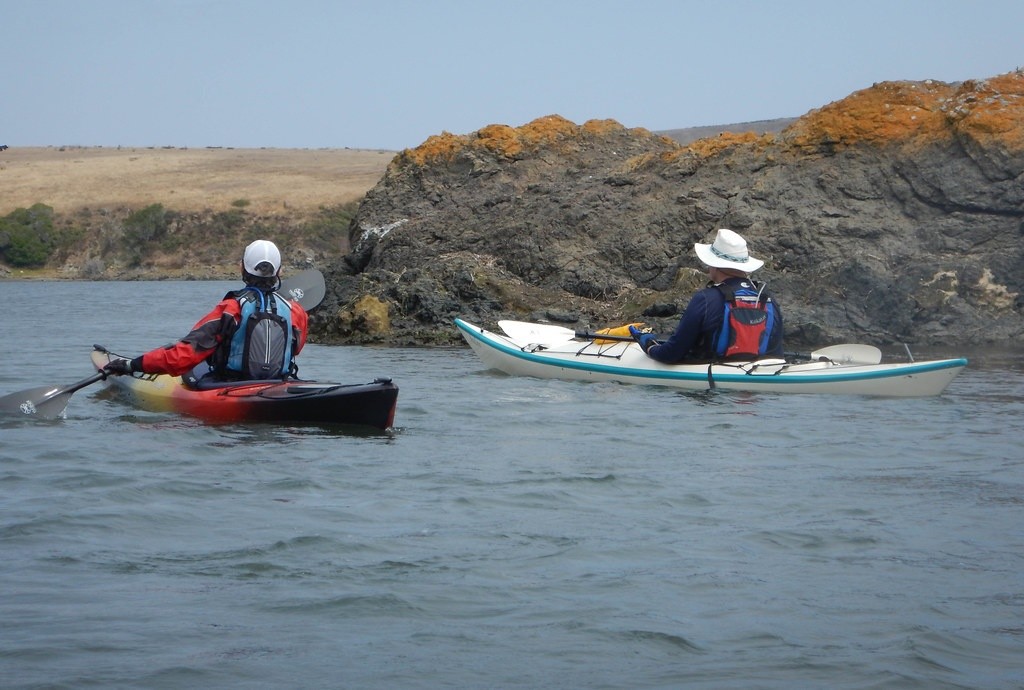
[0,265,329,427]
[574,330,884,365]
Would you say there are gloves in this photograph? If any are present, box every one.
[103,358,133,376]
[629,325,658,355]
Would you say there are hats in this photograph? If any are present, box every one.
[243,240,281,277]
[694,228,764,273]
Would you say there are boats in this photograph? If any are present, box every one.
[452,313,969,395]
[88,341,398,432]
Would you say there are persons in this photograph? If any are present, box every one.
[630,228,784,364]
[104,239,308,380]
